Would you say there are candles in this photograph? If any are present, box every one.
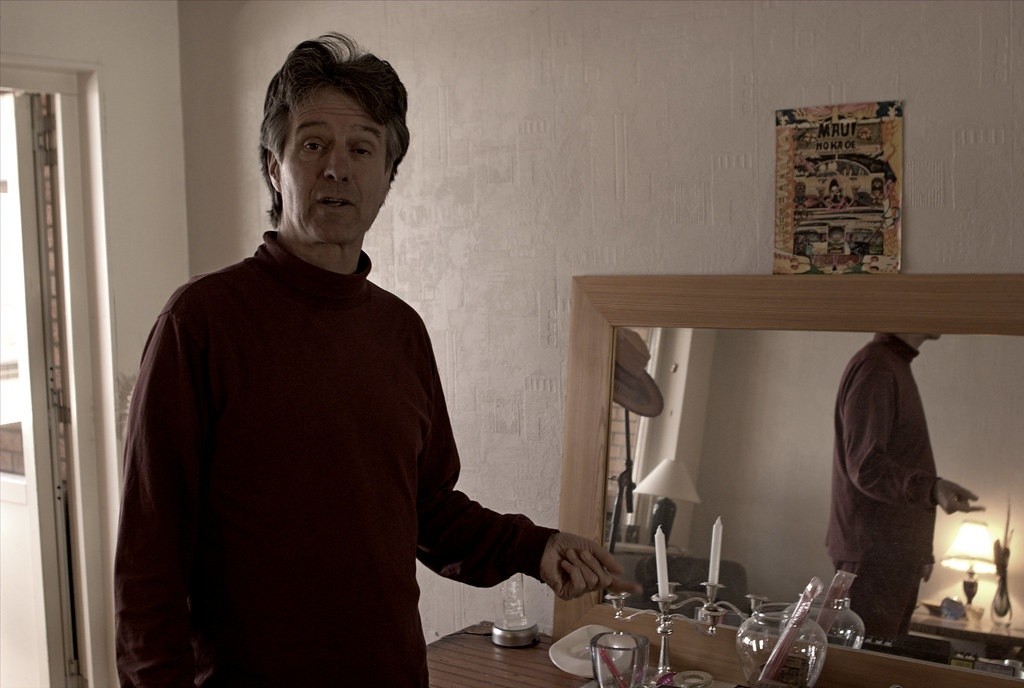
[706,516,724,583]
[652,522,670,598]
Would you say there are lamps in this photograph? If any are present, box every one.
[940,517,997,618]
[630,457,701,549]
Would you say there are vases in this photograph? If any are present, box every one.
[991,572,1013,628]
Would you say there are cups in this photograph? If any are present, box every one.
[590,630,649,688]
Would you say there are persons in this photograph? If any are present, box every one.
[824,333,985,639]
[114,34,641,688]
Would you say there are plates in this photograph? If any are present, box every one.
[549,625,636,679]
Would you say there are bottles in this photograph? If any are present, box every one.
[735,603,827,688]
[784,594,866,649]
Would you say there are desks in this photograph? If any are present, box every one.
[904,607,1023,672]
[424,620,764,688]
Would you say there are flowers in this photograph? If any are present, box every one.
[993,500,1014,573]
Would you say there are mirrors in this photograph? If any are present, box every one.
[550,274,1024,687]
